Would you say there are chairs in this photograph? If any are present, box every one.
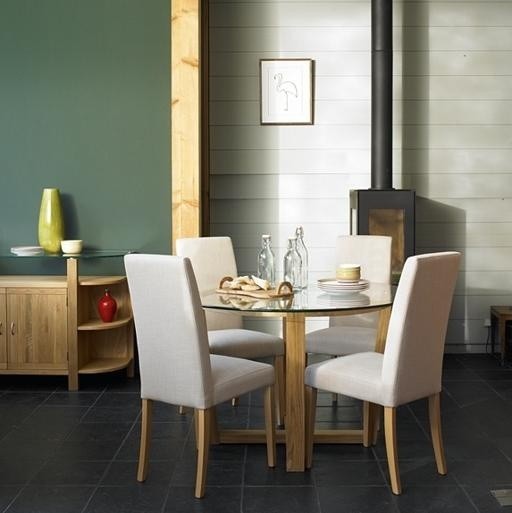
[175,236,284,454]
[300,235,394,449]
[303,252,462,495]
[123,252,279,500]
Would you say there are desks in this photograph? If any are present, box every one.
[196,279,396,474]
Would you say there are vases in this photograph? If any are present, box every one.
[97,287,117,323]
[36,187,65,258]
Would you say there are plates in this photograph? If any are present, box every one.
[317,277,371,297]
[10,246,44,255]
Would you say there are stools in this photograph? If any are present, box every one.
[490,304,512,367]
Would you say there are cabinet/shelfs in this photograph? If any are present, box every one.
[0,249,138,391]
[0,274,68,375]
[77,273,136,379]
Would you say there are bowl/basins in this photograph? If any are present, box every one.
[60,239,84,253]
[334,264,361,283]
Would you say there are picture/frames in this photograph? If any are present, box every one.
[258,58,315,126]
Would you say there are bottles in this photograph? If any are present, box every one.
[293,226,309,290]
[283,237,302,293]
[257,234,276,288]
